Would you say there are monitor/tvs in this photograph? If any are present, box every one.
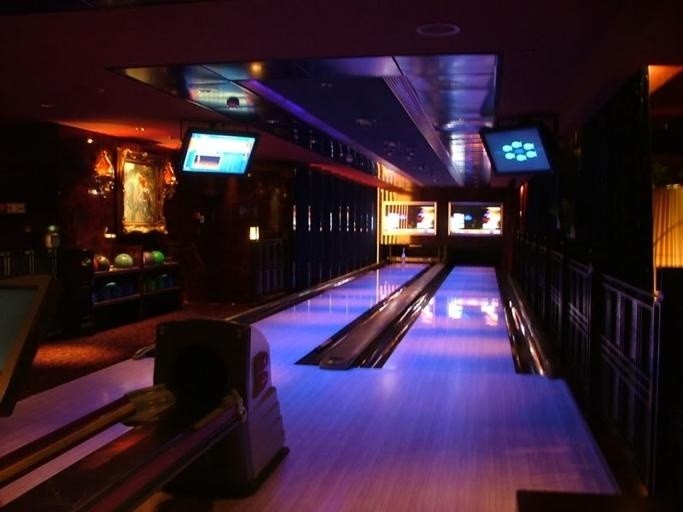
[476,123,553,184]
[1,275,60,418]
[181,128,256,180]
